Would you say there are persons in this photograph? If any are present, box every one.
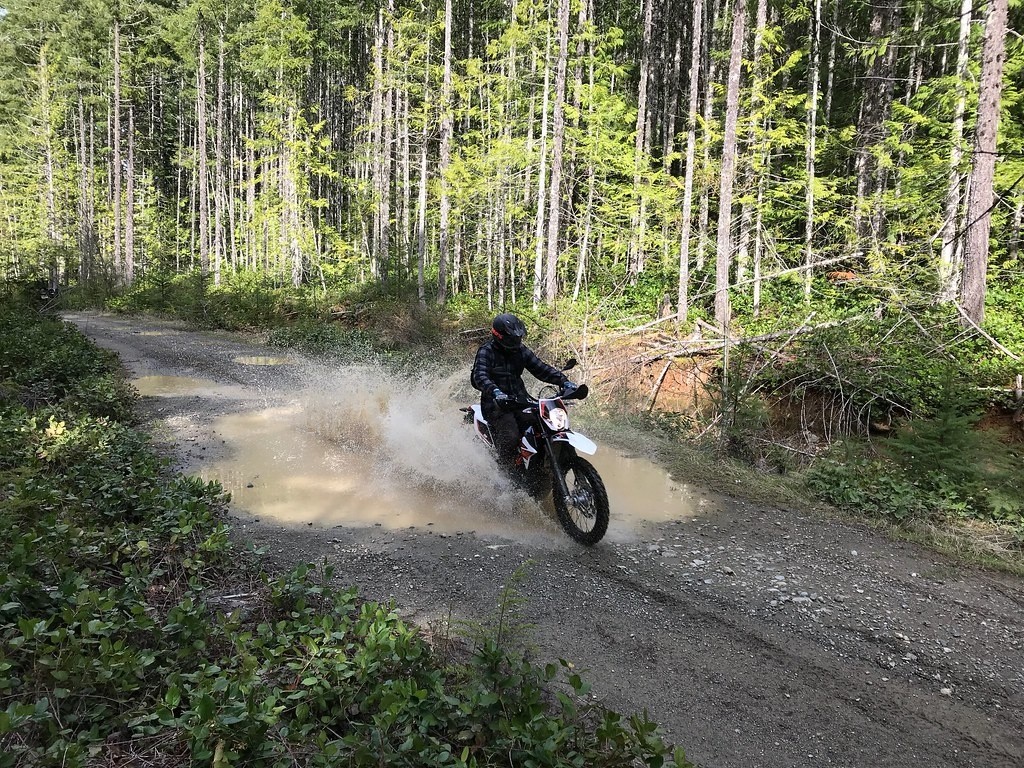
[470,314,577,514]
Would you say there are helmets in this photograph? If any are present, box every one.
[492,314,527,354]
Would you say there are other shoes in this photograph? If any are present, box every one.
[494,478,508,491]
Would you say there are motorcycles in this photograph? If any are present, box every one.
[459,359,610,546]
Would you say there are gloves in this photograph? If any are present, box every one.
[492,388,508,408]
[563,381,578,391]
